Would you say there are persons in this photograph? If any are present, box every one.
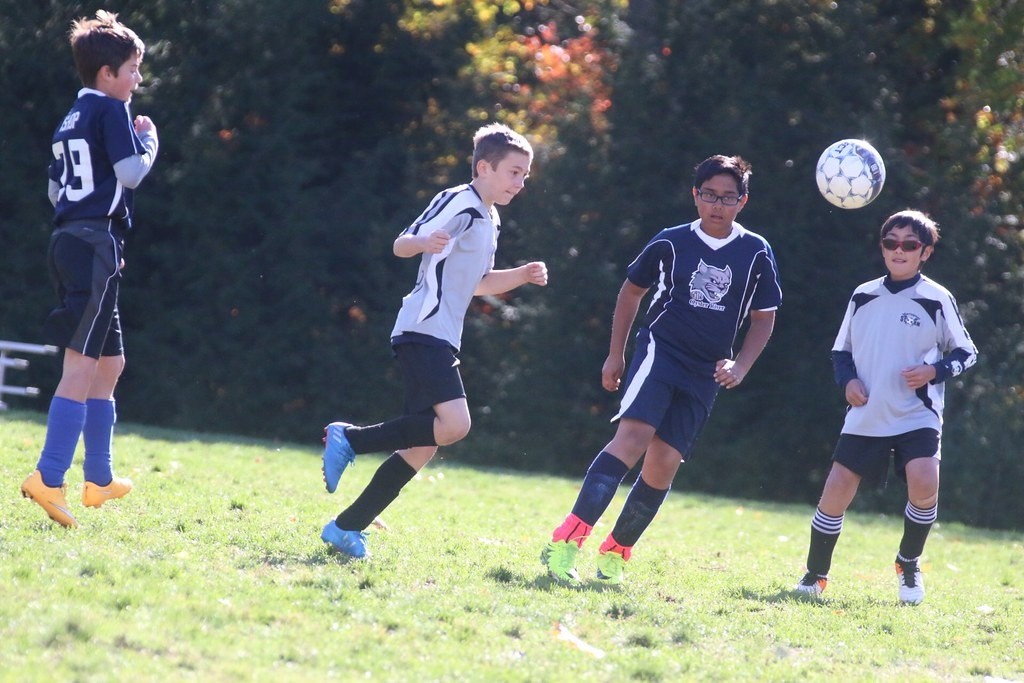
[319,123,548,558]
[20,8,158,534]
[794,212,979,605]
[540,154,782,588]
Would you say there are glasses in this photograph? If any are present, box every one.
[882,238,926,252]
[696,189,745,205]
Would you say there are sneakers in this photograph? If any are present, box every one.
[538,543,585,590]
[598,547,625,580]
[320,519,371,558]
[895,556,926,606]
[20,469,79,528]
[794,570,830,596]
[321,422,356,494]
[81,474,133,509]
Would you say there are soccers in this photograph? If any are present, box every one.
[815,137,888,210]
